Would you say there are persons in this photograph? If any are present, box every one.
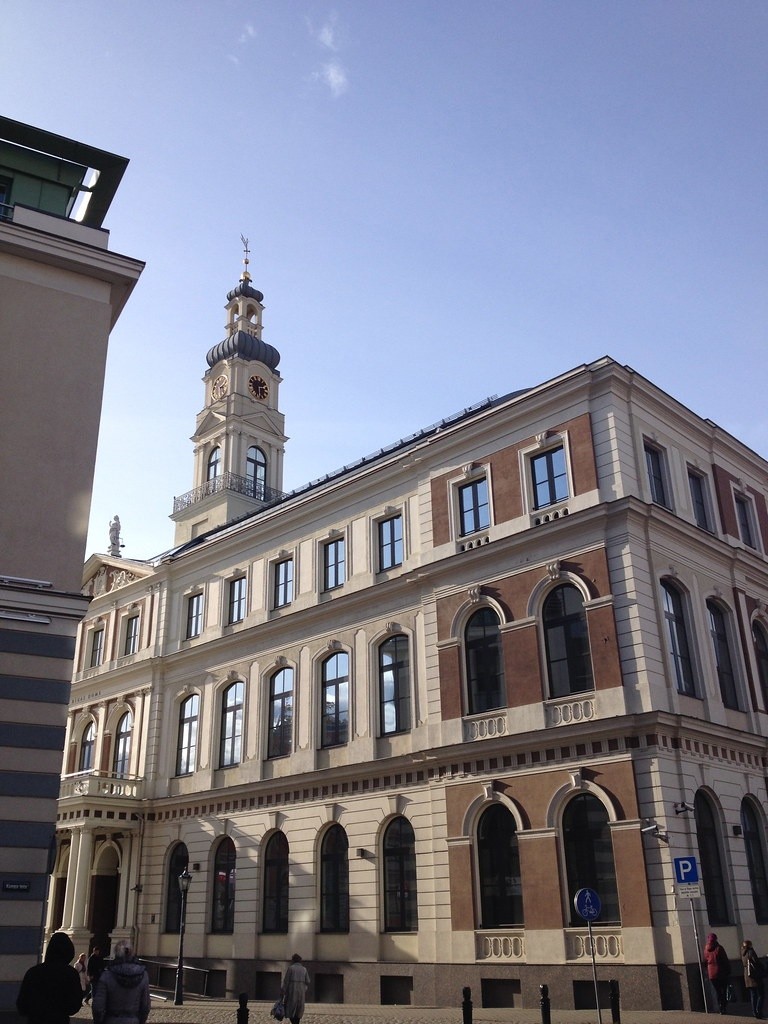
[279,953,310,1024]
[92,940,151,1024]
[704,933,731,1015]
[74,953,90,1007]
[16,932,83,1024]
[84,947,104,1006]
[741,940,764,1018]
[108,515,121,549]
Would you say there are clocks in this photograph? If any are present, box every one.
[248,375,270,401]
[211,374,229,400]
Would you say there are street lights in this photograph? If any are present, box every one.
[173,866,194,1006]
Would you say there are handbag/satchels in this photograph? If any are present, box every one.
[748,949,762,977]
[726,977,736,1002]
[271,997,285,1021]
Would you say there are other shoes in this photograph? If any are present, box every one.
[85,1000,90,1005]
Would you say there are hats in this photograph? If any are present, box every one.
[293,954,301,962]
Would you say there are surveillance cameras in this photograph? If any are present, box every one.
[680,802,696,812]
[640,825,658,833]
